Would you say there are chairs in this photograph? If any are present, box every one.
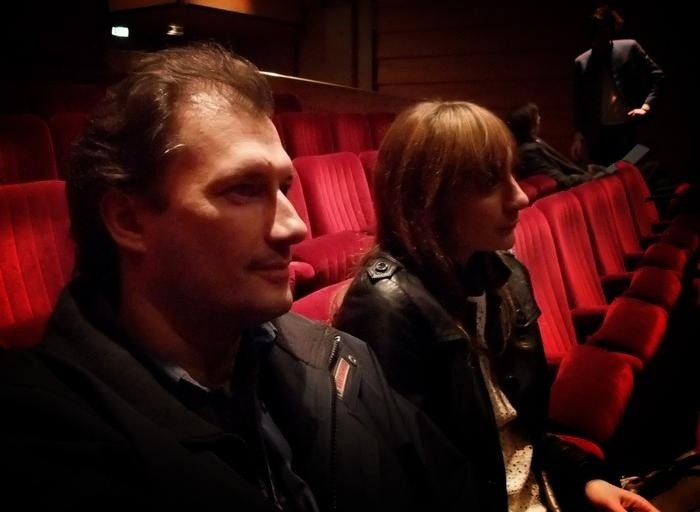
[0,78,700,511]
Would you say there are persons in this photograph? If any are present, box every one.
[507,100,613,189]
[570,3,666,215]
[328,99,662,512]
[1,40,481,512]
